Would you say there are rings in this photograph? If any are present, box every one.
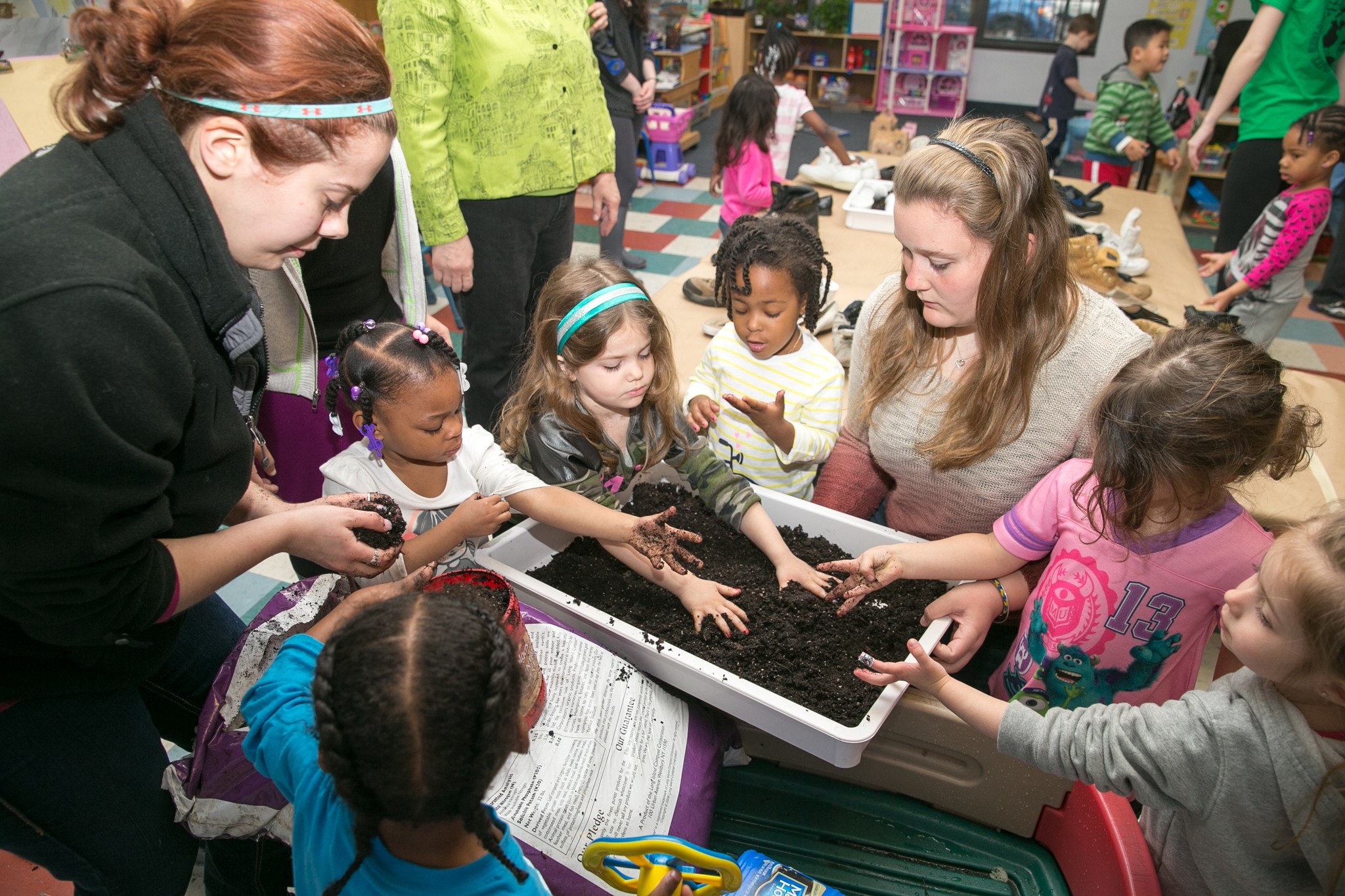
[365,548,380,567]
[367,492,372,501]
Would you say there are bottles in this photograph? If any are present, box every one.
[650,40,659,49]
[846,45,877,71]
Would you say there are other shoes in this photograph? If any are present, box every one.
[621,250,646,269]
[834,298,863,367]
[684,277,722,306]
[1309,297,1345,320]
[1065,233,1151,305]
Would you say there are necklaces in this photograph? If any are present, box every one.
[953,332,979,370]
[787,330,802,355]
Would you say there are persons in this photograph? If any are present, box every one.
[2,0,1345,896]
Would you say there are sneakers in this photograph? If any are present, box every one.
[1069,210,1155,276]
[1051,181,1111,217]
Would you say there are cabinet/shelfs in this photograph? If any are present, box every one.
[746,28,883,112]
[1156,114,1242,230]
[653,10,756,125]
[876,1,976,117]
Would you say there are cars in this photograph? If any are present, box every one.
[946,0,1061,41]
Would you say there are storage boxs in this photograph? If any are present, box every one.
[707,761,1074,896]
[842,177,894,231]
[475,458,977,768]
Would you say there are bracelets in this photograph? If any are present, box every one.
[988,579,1007,617]
[992,580,1009,623]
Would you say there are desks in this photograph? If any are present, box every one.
[652,145,1344,530]
